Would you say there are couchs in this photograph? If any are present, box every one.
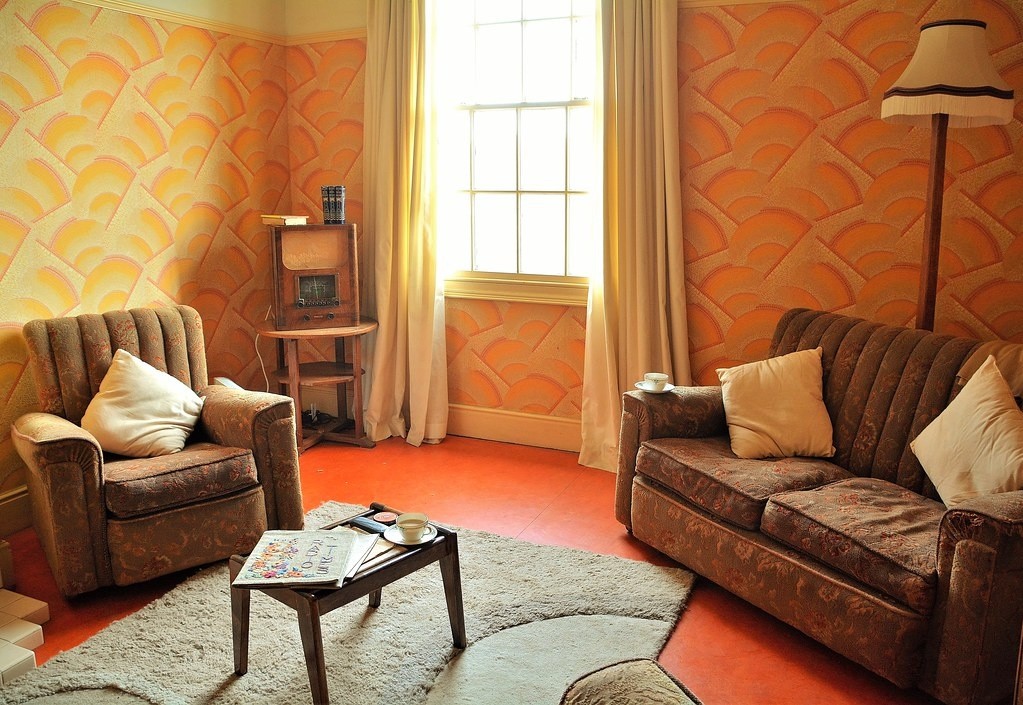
[614,307,1023,705]
[8,304,307,599]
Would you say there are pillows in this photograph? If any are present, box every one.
[80,347,208,457]
[713,346,837,459]
[908,353,1023,509]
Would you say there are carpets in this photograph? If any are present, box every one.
[0,498,695,705]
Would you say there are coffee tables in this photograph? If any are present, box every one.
[228,503,467,705]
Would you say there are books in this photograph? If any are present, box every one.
[260,214,310,225]
[231,525,407,591]
[320,185,346,224]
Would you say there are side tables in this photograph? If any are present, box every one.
[253,316,376,457]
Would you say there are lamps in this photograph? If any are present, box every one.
[882,20,1015,333]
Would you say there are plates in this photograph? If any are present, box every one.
[384,524,437,545]
[634,381,675,394]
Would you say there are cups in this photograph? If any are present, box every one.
[644,373,669,391]
[395,511,432,541]
